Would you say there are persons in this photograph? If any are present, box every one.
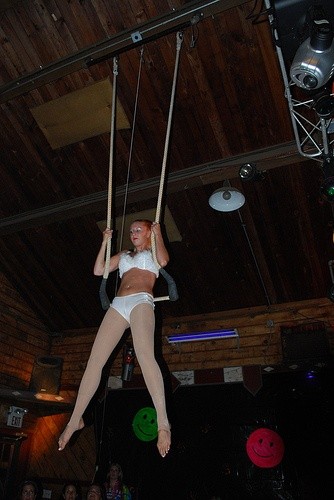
[86,484,103,500]
[63,482,78,500]
[58,219,171,458]
[18,482,37,500]
[104,463,132,500]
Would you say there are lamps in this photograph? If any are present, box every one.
[290,37,334,90]
[208,178,245,211]
[239,163,255,181]
